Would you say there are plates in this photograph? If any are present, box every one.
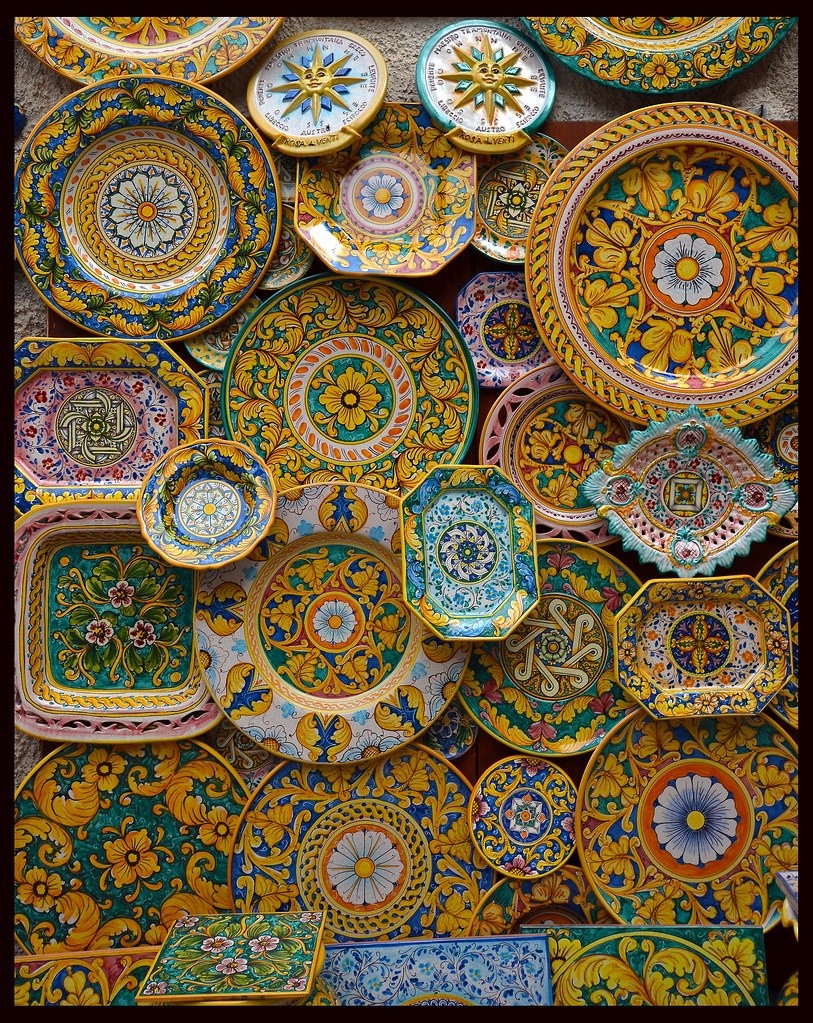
[15,16,800,1007]
